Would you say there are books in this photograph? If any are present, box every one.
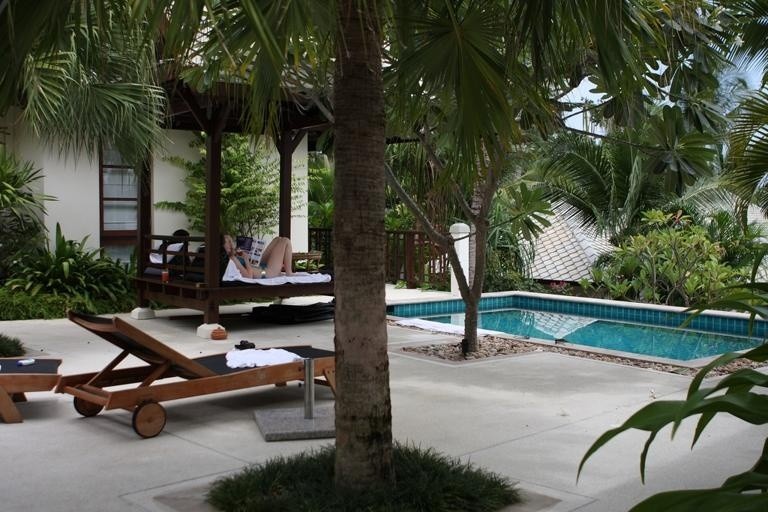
[236,236,265,267]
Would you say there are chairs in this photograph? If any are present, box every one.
[0,357,62,423]
[55,307,335,438]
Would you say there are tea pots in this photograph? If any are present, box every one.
[210,326,227,340]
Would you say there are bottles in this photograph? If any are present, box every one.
[16,359,35,366]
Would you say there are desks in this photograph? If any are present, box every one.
[292,249,322,274]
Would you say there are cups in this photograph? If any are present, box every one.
[161,268,169,284]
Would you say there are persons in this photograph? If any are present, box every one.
[157,229,196,263]
[220,234,293,277]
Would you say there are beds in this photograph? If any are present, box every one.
[127,229,335,340]
